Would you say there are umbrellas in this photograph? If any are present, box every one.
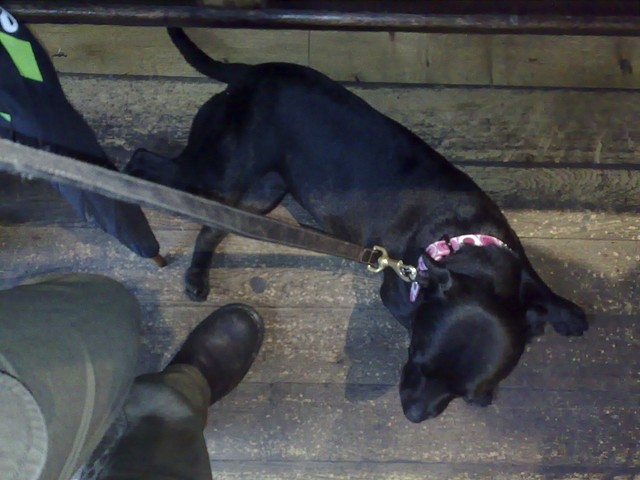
[0,8,167,268]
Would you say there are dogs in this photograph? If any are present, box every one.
[121,27,588,424]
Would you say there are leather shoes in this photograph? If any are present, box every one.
[160,303,265,409]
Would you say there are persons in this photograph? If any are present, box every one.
[0,271,264,479]
